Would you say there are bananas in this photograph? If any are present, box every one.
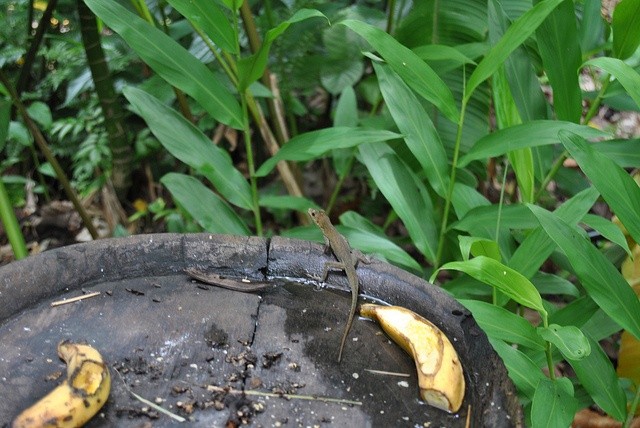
[361,303,467,415]
[11,340,111,428]
